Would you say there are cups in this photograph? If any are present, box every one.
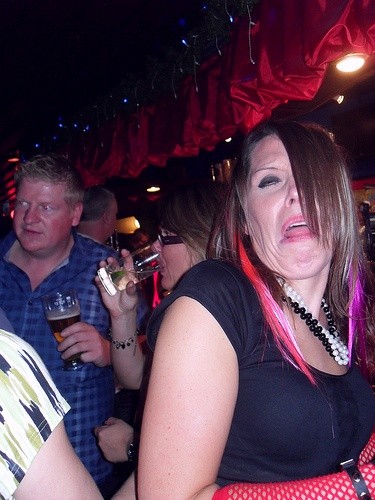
[97,242,164,297]
[106,224,119,252]
[41,289,89,370]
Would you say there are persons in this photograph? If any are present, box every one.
[94,180,230,463]
[0,151,148,500]
[138,116,375,500]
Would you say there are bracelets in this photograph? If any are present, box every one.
[128,441,136,463]
[341,458,373,500]
[106,328,139,349]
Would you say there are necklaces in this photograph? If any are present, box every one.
[274,277,350,366]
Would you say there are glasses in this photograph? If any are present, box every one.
[158,227,184,246]
